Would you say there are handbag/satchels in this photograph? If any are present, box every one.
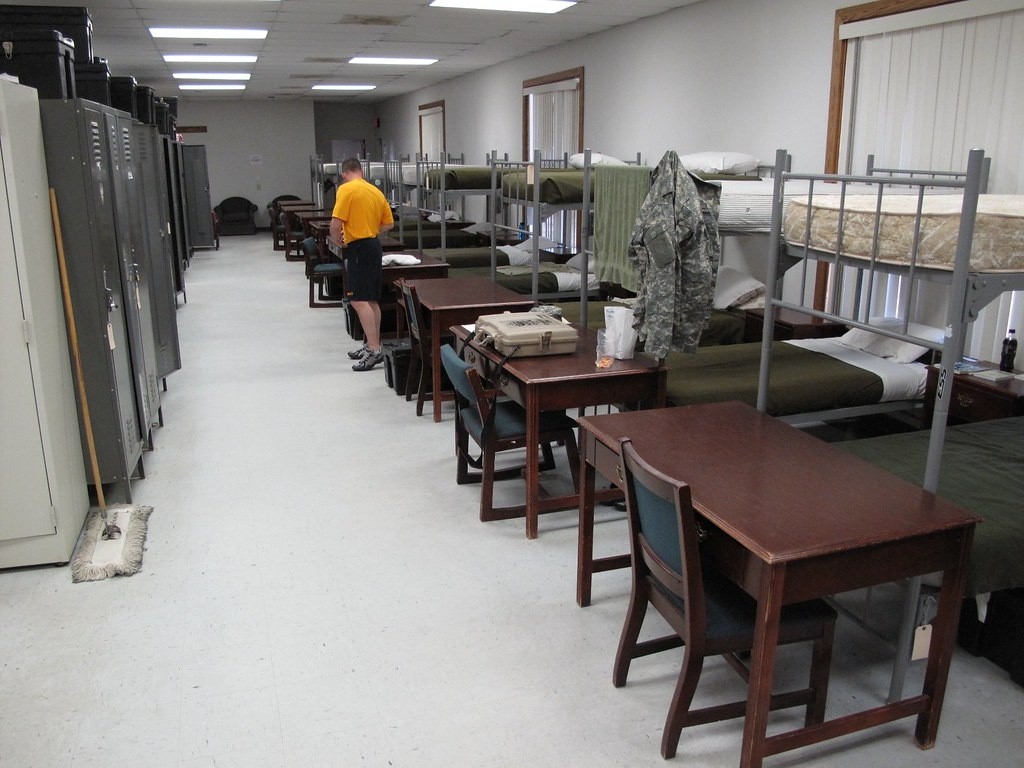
[604,306,640,358]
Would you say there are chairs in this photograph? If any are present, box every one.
[298,216,319,280]
[279,211,306,262]
[340,260,363,340]
[440,343,581,523]
[300,237,346,308]
[612,435,839,760]
[398,277,456,417]
[267,203,297,251]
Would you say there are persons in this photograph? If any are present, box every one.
[330,157,394,371]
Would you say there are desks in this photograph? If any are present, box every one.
[357,250,451,341]
[326,233,407,296]
[308,220,331,259]
[293,211,333,239]
[277,200,316,212]
[449,319,670,540]
[393,274,529,423]
[576,400,985,768]
[280,204,325,229]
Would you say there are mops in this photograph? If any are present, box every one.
[46,186,154,583]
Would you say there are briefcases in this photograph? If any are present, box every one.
[474,311,579,356]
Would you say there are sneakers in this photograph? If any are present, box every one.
[352,345,383,371]
[348,344,382,359]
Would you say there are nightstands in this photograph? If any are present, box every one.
[920,359,1024,428]
[744,306,850,343]
[476,231,523,247]
[538,247,582,265]
[598,282,637,301]
[440,219,476,230]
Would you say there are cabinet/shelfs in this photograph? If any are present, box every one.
[0,76,214,570]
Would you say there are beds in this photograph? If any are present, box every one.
[307,147,1024,710]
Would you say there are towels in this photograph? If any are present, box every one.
[593,164,651,293]
[382,254,421,266]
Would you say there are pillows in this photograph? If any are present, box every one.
[395,206,416,215]
[736,293,766,311]
[426,211,460,223]
[566,252,595,274]
[514,235,559,253]
[568,153,627,170]
[679,151,761,175]
[712,265,767,312]
[831,315,946,364]
[460,222,503,234]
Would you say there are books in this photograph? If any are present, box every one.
[974,369,1015,381]
[933,363,989,375]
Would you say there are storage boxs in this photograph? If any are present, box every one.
[0,4,179,140]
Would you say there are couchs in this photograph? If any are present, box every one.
[270,195,303,232]
[214,197,257,237]
